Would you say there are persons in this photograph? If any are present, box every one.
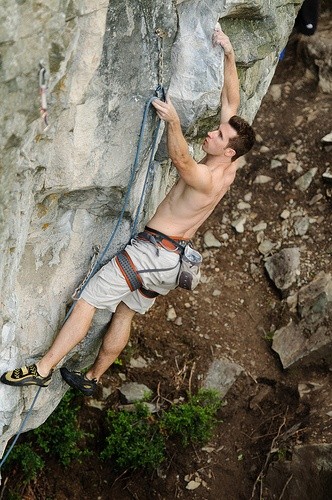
[1,29,256,392]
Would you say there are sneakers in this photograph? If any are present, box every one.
[61,367,96,396]
[0,364,53,387]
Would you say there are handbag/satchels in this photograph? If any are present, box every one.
[177,246,202,292]
